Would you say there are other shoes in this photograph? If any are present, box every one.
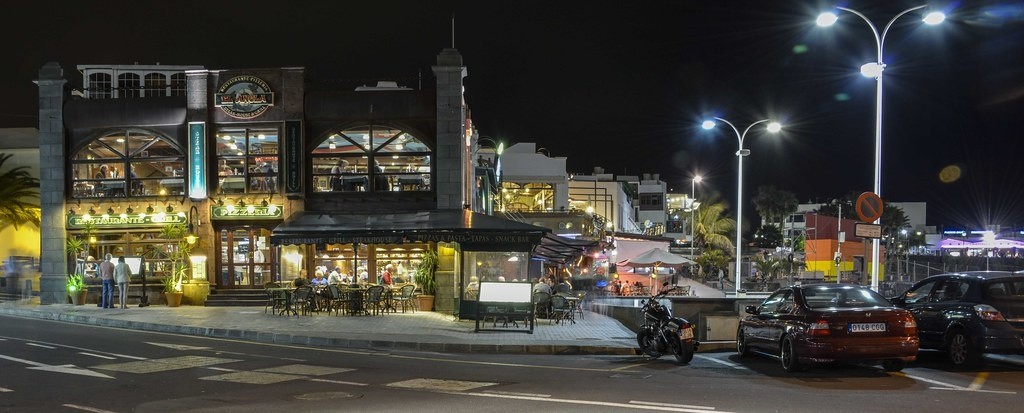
[103,306,108,309]
[311,308,318,312]
[124,305,130,309]
[120,306,123,309]
[317,308,322,312]
[327,307,332,311]
[109,306,118,309]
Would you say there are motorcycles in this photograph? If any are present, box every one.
[637,281,700,363]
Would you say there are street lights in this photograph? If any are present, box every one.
[816,3,947,294]
[691,175,703,275]
[701,115,783,297]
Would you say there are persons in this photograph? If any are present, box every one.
[94,164,140,196]
[291,259,405,311]
[217,167,265,194]
[220,244,265,285]
[608,280,640,297]
[466,275,570,318]
[100,252,118,308]
[718,266,725,291]
[330,159,383,191]
[113,256,132,309]
[84,255,100,292]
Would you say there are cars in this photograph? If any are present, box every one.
[889,271,1024,371]
[737,281,921,375]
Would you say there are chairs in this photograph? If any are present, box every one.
[464,285,586,328]
[74,181,146,197]
[608,285,691,298]
[264,281,417,318]
[391,177,404,191]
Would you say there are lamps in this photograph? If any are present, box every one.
[88,205,102,216]
[146,204,158,214]
[107,205,121,215]
[217,197,227,206]
[69,207,81,215]
[261,197,270,207]
[126,205,139,215]
[166,203,178,213]
[184,205,201,244]
[238,198,248,208]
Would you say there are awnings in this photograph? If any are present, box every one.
[529,231,598,268]
[268,207,553,253]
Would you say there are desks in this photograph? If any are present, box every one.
[339,176,368,192]
[267,288,306,318]
[340,288,371,317]
[381,286,402,313]
[100,181,126,197]
[398,175,422,190]
[221,177,246,194]
[74,184,97,197]
[306,284,327,312]
[160,179,184,195]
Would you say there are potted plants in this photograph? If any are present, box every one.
[413,248,437,311]
[152,222,191,308]
[66,220,97,305]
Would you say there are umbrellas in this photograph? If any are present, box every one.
[614,248,699,299]
